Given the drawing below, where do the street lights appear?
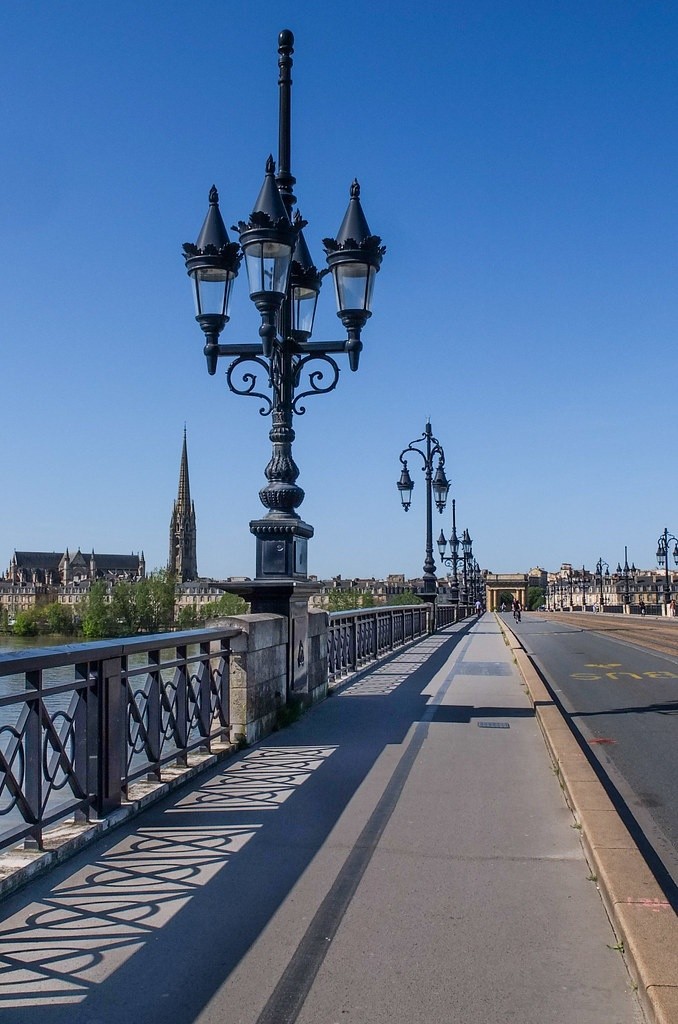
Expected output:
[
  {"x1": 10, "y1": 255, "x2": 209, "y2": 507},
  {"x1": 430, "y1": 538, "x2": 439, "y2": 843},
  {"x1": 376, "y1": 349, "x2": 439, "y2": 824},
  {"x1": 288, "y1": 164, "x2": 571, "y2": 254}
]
[
  {"x1": 656, "y1": 527, "x2": 678, "y2": 601},
  {"x1": 566, "y1": 567, "x2": 576, "y2": 605},
  {"x1": 576, "y1": 565, "x2": 592, "y2": 602},
  {"x1": 616, "y1": 546, "x2": 640, "y2": 601},
  {"x1": 595, "y1": 557, "x2": 611, "y2": 603},
  {"x1": 436, "y1": 496, "x2": 474, "y2": 599},
  {"x1": 396, "y1": 413, "x2": 453, "y2": 594},
  {"x1": 454, "y1": 528, "x2": 473, "y2": 600},
  {"x1": 180, "y1": 26, "x2": 395, "y2": 581}
]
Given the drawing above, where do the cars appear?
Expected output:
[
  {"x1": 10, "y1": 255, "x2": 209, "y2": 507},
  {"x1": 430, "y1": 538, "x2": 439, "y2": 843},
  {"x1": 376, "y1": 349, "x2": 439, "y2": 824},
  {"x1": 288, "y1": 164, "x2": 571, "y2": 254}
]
[{"x1": 537, "y1": 606, "x2": 546, "y2": 612}]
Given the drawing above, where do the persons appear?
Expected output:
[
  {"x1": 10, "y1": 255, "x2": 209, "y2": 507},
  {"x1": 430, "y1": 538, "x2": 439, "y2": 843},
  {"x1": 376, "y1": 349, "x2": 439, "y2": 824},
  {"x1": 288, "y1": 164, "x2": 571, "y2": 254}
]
[
  {"x1": 502, "y1": 603, "x2": 506, "y2": 614},
  {"x1": 474, "y1": 599, "x2": 482, "y2": 618},
  {"x1": 511, "y1": 598, "x2": 521, "y2": 622},
  {"x1": 639, "y1": 598, "x2": 646, "y2": 614},
  {"x1": 669, "y1": 600, "x2": 676, "y2": 617}
]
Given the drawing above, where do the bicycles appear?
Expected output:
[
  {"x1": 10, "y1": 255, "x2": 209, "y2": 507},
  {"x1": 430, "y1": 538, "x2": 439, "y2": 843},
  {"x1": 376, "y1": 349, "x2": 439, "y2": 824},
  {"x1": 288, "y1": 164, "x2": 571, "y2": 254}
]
[{"x1": 514, "y1": 609, "x2": 521, "y2": 624}]
